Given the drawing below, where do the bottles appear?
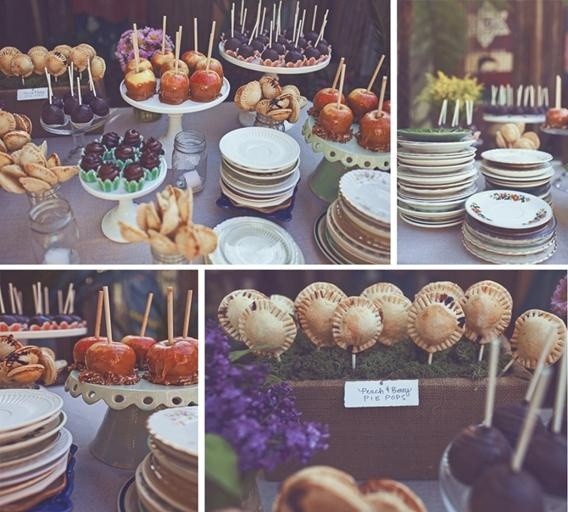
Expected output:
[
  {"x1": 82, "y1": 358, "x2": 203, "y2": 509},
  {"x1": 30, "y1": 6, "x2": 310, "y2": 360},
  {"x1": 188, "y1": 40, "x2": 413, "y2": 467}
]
[
  {"x1": 26, "y1": 196, "x2": 84, "y2": 263},
  {"x1": 171, "y1": 127, "x2": 209, "y2": 196}
]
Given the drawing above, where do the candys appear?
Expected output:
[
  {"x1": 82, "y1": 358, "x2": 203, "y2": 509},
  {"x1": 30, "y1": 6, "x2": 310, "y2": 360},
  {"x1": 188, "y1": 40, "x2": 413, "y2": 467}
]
[
  {"x1": 1, "y1": 1, "x2": 391, "y2": 263},
  {"x1": 485, "y1": 75, "x2": 568, "y2": 149},
  {"x1": 0, "y1": 282, "x2": 198, "y2": 389},
  {"x1": 215, "y1": 278, "x2": 567, "y2": 511}
]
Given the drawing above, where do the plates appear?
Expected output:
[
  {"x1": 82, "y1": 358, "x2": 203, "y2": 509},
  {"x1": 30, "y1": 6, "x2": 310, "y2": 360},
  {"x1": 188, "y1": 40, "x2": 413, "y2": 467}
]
[
  {"x1": 215, "y1": 125, "x2": 392, "y2": 264},
  {"x1": 2, "y1": 388, "x2": 202, "y2": 512},
  {"x1": 399, "y1": 126, "x2": 560, "y2": 264}
]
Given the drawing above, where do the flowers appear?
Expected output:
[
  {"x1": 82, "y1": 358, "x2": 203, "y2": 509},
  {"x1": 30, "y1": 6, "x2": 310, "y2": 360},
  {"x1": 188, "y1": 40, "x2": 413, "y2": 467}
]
[
  {"x1": 115, "y1": 27, "x2": 174, "y2": 69},
  {"x1": 205, "y1": 316, "x2": 336, "y2": 497},
  {"x1": 551, "y1": 273, "x2": 568, "y2": 316},
  {"x1": 412, "y1": 72, "x2": 485, "y2": 128}
]
